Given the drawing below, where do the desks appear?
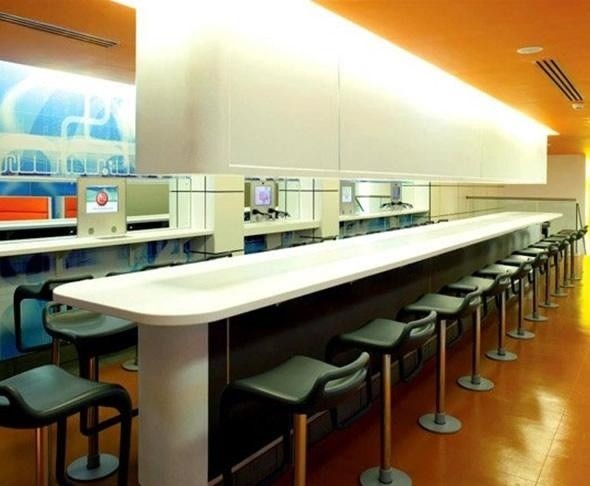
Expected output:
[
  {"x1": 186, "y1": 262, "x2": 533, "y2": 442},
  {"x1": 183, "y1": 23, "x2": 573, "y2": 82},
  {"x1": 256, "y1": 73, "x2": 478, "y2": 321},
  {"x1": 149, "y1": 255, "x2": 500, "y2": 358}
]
[{"x1": 51, "y1": 211, "x2": 566, "y2": 486}]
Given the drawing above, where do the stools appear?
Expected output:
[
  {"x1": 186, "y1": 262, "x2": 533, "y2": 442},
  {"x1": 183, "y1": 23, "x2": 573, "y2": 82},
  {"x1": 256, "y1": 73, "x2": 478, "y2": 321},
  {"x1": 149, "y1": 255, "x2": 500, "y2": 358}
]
[
  {"x1": 397, "y1": 225, "x2": 587, "y2": 434},
  {"x1": 220, "y1": 351, "x2": 371, "y2": 483},
  {"x1": 326, "y1": 311, "x2": 438, "y2": 483}
]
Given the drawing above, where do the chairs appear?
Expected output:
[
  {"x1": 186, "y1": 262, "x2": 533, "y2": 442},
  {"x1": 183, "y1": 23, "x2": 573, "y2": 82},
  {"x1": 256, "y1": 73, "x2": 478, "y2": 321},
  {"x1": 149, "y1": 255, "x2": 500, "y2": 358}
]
[{"x1": 0, "y1": 273, "x2": 140, "y2": 486}]
